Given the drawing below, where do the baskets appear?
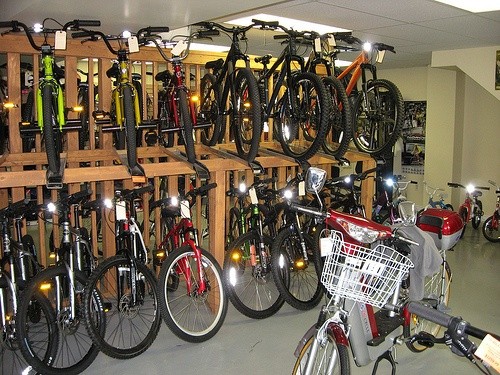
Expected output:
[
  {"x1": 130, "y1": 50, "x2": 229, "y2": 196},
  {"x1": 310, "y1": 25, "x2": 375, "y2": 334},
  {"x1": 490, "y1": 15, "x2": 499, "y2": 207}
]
[{"x1": 318, "y1": 229, "x2": 414, "y2": 309}]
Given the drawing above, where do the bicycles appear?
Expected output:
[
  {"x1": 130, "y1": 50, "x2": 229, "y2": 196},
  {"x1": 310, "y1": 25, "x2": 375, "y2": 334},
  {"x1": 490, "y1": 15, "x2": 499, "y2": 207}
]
[
  {"x1": 151, "y1": 183, "x2": 229, "y2": 343},
  {"x1": 337, "y1": 32, "x2": 405, "y2": 157},
  {"x1": 3, "y1": 165, "x2": 401, "y2": 370},
  {"x1": 222, "y1": 176, "x2": 290, "y2": 319},
  {"x1": 392, "y1": 179, "x2": 417, "y2": 201},
  {"x1": 125, "y1": 28, "x2": 221, "y2": 161},
  {"x1": 373, "y1": 175, "x2": 401, "y2": 226},
  {"x1": 483, "y1": 180, "x2": 500, "y2": 242},
  {"x1": 83, "y1": 183, "x2": 161, "y2": 360},
  {"x1": 0, "y1": 199, "x2": 59, "y2": 375},
  {"x1": 417, "y1": 181, "x2": 455, "y2": 216},
  {"x1": 255, "y1": 18, "x2": 330, "y2": 161},
  {"x1": 274, "y1": 32, "x2": 353, "y2": 157},
  {"x1": 447, "y1": 179, "x2": 490, "y2": 239},
  {"x1": 15, "y1": 188, "x2": 106, "y2": 375},
  {"x1": 195, "y1": 18, "x2": 279, "y2": 161},
  {"x1": 1, "y1": 18, "x2": 101, "y2": 174},
  {"x1": 272, "y1": 171, "x2": 326, "y2": 310},
  {"x1": 314, "y1": 167, "x2": 380, "y2": 303},
  {"x1": 70, "y1": 26, "x2": 169, "y2": 168}
]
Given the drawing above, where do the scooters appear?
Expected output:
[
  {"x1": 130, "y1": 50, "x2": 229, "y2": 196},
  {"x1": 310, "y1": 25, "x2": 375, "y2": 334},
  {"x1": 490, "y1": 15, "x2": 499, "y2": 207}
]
[
  {"x1": 290, "y1": 166, "x2": 464, "y2": 375},
  {"x1": 408, "y1": 301, "x2": 500, "y2": 375}
]
